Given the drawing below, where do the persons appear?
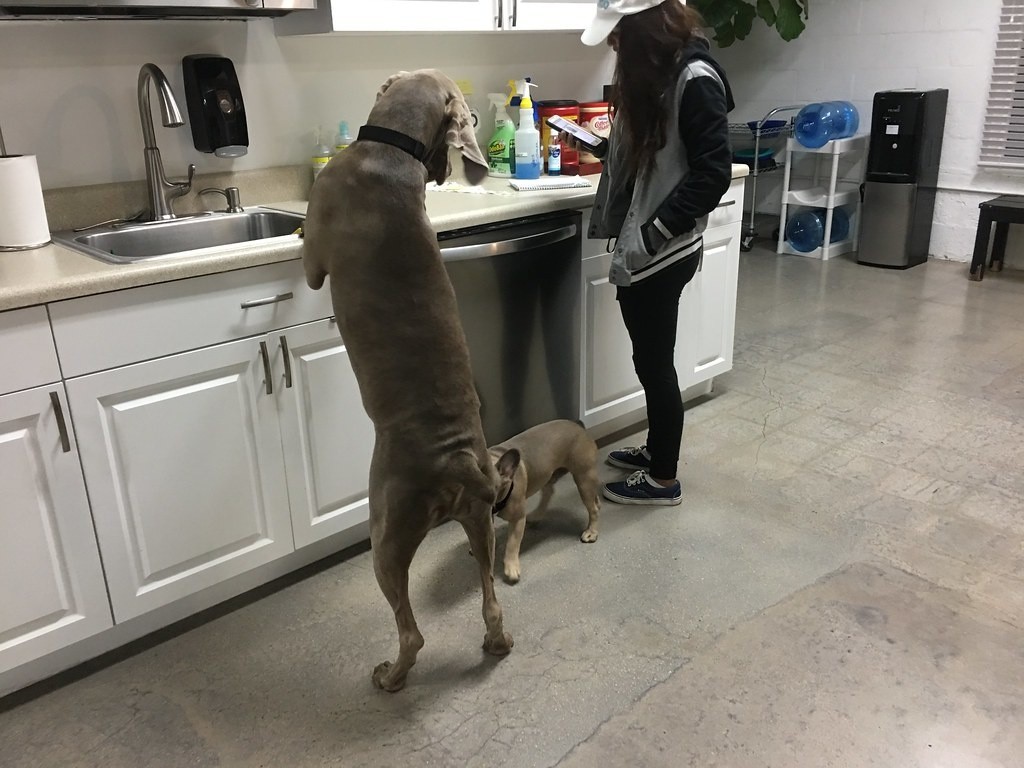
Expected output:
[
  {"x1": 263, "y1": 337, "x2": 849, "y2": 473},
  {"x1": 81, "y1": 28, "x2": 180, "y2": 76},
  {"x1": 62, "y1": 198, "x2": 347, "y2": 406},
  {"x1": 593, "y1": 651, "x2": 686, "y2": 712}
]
[{"x1": 577, "y1": 0, "x2": 734, "y2": 508}]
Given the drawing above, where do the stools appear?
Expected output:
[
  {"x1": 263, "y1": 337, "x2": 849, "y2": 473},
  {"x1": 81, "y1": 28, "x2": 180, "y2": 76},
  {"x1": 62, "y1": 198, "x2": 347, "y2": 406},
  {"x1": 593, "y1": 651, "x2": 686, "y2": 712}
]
[{"x1": 967, "y1": 192, "x2": 1024, "y2": 281}]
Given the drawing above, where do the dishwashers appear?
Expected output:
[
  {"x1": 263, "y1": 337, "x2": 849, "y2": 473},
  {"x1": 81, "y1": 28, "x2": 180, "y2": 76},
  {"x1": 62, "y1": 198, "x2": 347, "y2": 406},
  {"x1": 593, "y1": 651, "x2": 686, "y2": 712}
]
[{"x1": 428, "y1": 207, "x2": 587, "y2": 444}]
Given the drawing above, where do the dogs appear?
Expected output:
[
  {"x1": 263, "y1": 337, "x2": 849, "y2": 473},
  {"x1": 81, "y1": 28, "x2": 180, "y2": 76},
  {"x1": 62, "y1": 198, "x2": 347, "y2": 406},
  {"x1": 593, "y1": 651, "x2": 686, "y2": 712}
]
[
  {"x1": 299, "y1": 67, "x2": 519, "y2": 693},
  {"x1": 468, "y1": 418, "x2": 601, "y2": 583}
]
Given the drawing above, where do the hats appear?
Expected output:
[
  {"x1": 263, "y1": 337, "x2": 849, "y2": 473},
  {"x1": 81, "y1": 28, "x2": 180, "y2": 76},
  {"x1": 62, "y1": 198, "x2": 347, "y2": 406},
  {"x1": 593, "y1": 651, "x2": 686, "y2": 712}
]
[{"x1": 579, "y1": 1, "x2": 673, "y2": 46}]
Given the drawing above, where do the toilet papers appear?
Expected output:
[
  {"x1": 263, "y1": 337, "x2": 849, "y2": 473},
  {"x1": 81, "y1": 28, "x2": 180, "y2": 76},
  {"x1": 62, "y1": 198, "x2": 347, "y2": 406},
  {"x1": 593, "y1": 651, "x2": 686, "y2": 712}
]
[{"x1": 0, "y1": 153, "x2": 53, "y2": 253}]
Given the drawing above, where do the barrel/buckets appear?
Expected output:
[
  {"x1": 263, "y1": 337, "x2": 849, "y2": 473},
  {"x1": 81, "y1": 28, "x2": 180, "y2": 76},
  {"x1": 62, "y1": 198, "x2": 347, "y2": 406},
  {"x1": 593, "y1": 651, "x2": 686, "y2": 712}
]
[{"x1": 535, "y1": 97, "x2": 615, "y2": 170}]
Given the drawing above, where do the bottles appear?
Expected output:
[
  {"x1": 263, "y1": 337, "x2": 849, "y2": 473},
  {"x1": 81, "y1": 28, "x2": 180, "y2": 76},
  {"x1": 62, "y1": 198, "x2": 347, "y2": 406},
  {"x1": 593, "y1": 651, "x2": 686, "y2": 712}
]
[
  {"x1": 793, "y1": 100, "x2": 860, "y2": 149},
  {"x1": 787, "y1": 207, "x2": 850, "y2": 253}
]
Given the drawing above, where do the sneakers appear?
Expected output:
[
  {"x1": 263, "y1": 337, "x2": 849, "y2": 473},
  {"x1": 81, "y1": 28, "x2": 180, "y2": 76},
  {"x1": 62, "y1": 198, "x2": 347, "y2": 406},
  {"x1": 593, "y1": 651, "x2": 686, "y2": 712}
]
[
  {"x1": 599, "y1": 472, "x2": 683, "y2": 507},
  {"x1": 606, "y1": 445, "x2": 652, "y2": 471}
]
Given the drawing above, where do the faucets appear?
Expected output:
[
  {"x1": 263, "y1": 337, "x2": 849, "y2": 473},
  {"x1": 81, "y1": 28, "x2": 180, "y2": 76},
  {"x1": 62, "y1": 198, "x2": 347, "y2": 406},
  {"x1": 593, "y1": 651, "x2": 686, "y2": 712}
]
[{"x1": 136, "y1": 62, "x2": 186, "y2": 222}]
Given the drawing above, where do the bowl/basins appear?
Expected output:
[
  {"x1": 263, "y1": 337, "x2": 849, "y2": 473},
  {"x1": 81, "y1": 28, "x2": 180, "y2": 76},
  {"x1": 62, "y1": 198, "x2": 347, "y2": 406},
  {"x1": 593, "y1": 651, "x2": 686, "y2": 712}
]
[{"x1": 747, "y1": 117, "x2": 787, "y2": 138}]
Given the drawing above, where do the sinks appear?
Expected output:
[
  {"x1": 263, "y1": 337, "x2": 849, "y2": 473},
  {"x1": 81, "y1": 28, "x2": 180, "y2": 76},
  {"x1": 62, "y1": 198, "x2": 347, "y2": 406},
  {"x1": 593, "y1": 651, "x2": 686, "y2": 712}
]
[{"x1": 50, "y1": 205, "x2": 313, "y2": 265}]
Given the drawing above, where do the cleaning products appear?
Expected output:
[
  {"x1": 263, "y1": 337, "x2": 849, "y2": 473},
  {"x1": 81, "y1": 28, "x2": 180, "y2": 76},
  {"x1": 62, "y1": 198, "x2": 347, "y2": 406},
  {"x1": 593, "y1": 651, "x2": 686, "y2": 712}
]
[{"x1": 309, "y1": 77, "x2": 562, "y2": 191}]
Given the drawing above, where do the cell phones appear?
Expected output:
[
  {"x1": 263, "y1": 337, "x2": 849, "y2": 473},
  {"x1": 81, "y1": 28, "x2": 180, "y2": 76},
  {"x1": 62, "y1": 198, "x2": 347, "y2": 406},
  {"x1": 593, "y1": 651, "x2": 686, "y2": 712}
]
[{"x1": 545, "y1": 115, "x2": 602, "y2": 151}]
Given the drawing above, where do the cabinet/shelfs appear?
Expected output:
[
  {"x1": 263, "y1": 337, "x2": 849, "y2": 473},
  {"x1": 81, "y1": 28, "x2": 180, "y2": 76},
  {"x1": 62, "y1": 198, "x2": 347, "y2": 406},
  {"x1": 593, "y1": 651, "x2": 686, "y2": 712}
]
[
  {"x1": 776, "y1": 125, "x2": 870, "y2": 263},
  {"x1": 272, "y1": 2, "x2": 684, "y2": 43},
  {"x1": 725, "y1": 104, "x2": 807, "y2": 252},
  {"x1": 0, "y1": 150, "x2": 757, "y2": 707}
]
